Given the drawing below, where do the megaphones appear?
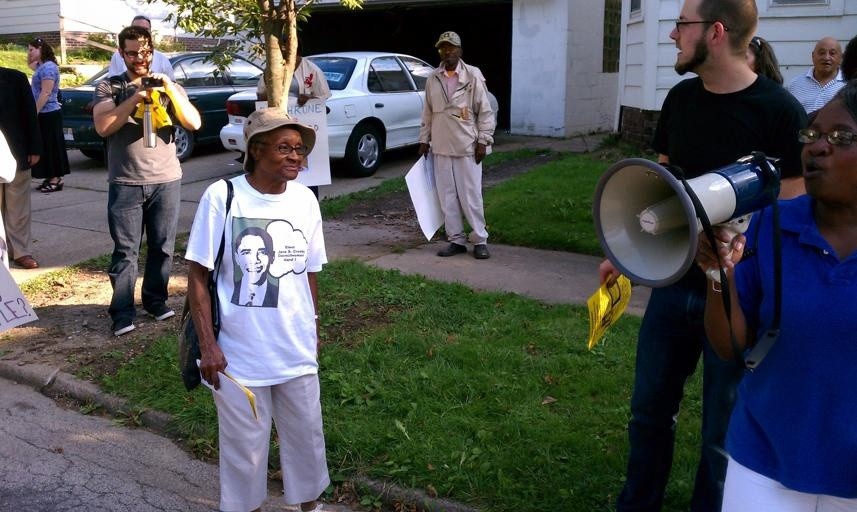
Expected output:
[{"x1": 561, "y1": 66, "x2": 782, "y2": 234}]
[{"x1": 593, "y1": 151, "x2": 781, "y2": 288}]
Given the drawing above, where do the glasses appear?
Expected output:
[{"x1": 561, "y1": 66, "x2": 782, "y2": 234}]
[
  {"x1": 255, "y1": 139, "x2": 308, "y2": 156},
  {"x1": 797, "y1": 127, "x2": 857, "y2": 149},
  {"x1": 121, "y1": 48, "x2": 154, "y2": 58},
  {"x1": 438, "y1": 47, "x2": 460, "y2": 54},
  {"x1": 674, "y1": 17, "x2": 729, "y2": 34}
]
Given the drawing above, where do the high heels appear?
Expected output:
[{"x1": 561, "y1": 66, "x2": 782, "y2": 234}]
[
  {"x1": 41, "y1": 178, "x2": 64, "y2": 193},
  {"x1": 35, "y1": 179, "x2": 51, "y2": 191}
]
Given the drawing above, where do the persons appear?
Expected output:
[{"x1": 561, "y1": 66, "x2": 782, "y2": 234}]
[
  {"x1": 27, "y1": 37, "x2": 71, "y2": 193},
  {"x1": 418, "y1": 30, "x2": 497, "y2": 258},
  {"x1": 600, "y1": 2, "x2": 808, "y2": 512},
  {"x1": 182, "y1": 106, "x2": 332, "y2": 512},
  {"x1": 0, "y1": 66, "x2": 43, "y2": 269},
  {"x1": 91, "y1": 26, "x2": 203, "y2": 336},
  {"x1": 698, "y1": 87, "x2": 856, "y2": 511},
  {"x1": 746, "y1": 36, "x2": 856, "y2": 119},
  {"x1": 231, "y1": 226, "x2": 279, "y2": 307},
  {"x1": 256, "y1": 31, "x2": 332, "y2": 202},
  {"x1": 108, "y1": 16, "x2": 176, "y2": 82},
  {"x1": 0, "y1": 129, "x2": 17, "y2": 269}
]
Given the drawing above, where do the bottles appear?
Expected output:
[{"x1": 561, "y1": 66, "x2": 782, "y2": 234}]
[{"x1": 142, "y1": 96, "x2": 157, "y2": 148}]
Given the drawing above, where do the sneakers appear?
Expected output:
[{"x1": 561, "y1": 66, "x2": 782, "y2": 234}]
[
  {"x1": 472, "y1": 243, "x2": 491, "y2": 259},
  {"x1": 144, "y1": 302, "x2": 176, "y2": 321},
  {"x1": 110, "y1": 318, "x2": 137, "y2": 337},
  {"x1": 436, "y1": 242, "x2": 468, "y2": 257}
]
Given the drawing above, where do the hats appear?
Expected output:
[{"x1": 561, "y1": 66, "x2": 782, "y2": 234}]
[
  {"x1": 241, "y1": 106, "x2": 316, "y2": 174},
  {"x1": 435, "y1": 31, "x2": 462, "y2": 48}
]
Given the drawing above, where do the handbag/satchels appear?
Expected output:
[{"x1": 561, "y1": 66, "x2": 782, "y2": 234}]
[{"x1": 177, "y1": 276, "x2": 223, "y2": 393}]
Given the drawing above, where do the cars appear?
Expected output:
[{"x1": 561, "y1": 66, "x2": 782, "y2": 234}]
[
  {"x1": 54, "y1": 47, "x2": 264, "y2": 164},
  {"x1": 218, "y1": 50, "x2": 502, "y2": 180}
]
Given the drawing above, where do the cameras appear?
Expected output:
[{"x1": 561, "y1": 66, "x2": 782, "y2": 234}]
[{"x1": 142, "y1": 77, "x2": 163, "y2": 87}]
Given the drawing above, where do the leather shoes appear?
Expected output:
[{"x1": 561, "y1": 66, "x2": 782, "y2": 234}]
[{"x1": 14, "y1": 255, "x2": 39, "y2": 270}]
